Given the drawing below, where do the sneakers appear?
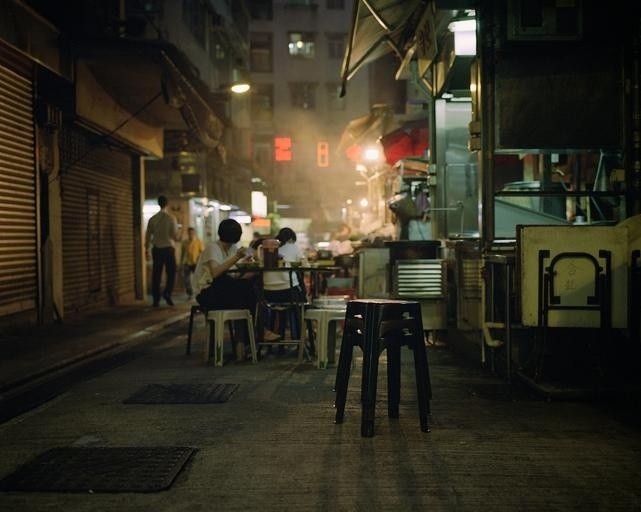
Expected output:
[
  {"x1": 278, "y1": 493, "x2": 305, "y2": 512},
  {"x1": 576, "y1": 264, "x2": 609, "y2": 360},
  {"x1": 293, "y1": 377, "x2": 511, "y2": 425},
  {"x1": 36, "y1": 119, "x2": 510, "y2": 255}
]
[
  {"x1": 257, "y1": 326, "x2": 281, "y2": 340},
  {"x1": 153, "y1": 291, "x2": 174, "y2": 306}
]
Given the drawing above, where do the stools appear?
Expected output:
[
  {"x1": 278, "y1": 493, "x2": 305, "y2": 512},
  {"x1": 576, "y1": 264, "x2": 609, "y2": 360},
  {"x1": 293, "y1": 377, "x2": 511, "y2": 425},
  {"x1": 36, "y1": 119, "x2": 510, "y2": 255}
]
[
  {"x1": 188, "y1": 294, "x2": 348, "y2": 371},
  {"x1": 332, "y1": 300, "x2": 434, "y2": 439}
]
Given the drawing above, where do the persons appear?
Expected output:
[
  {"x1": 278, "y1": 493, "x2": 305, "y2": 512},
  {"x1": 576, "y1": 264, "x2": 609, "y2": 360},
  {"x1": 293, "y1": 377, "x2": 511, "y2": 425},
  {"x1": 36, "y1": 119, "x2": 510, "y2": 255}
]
[
  {"x1": 179, "y1": 226, "x2": 202, "y2": 302},
  {"x1": 145, "y1": 195, "x2": 183, "y2": 308},
  {"x1": 191, "y1": 219, "x2": 304, "y2": 343},
  {"x1": 329, "y1": 224, "x2": 354, "y2": 260}
]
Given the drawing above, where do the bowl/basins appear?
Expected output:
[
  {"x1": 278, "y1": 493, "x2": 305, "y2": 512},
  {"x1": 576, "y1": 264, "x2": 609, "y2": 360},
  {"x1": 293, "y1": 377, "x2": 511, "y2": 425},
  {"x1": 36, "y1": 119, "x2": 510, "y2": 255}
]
[
  {"x1": 234, "y1": 262, "x2": 259, "y2": 270},
  {"x1": 289, "y1": 261, "x2": 301, "y2": 268}
]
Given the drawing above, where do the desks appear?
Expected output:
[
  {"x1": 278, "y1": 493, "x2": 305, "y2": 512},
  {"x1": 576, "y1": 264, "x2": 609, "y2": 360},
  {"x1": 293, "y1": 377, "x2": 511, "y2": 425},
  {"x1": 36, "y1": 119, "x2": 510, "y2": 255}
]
[{"x1": 228, "y1": 266, "x2": 336, "y2": 362}]
